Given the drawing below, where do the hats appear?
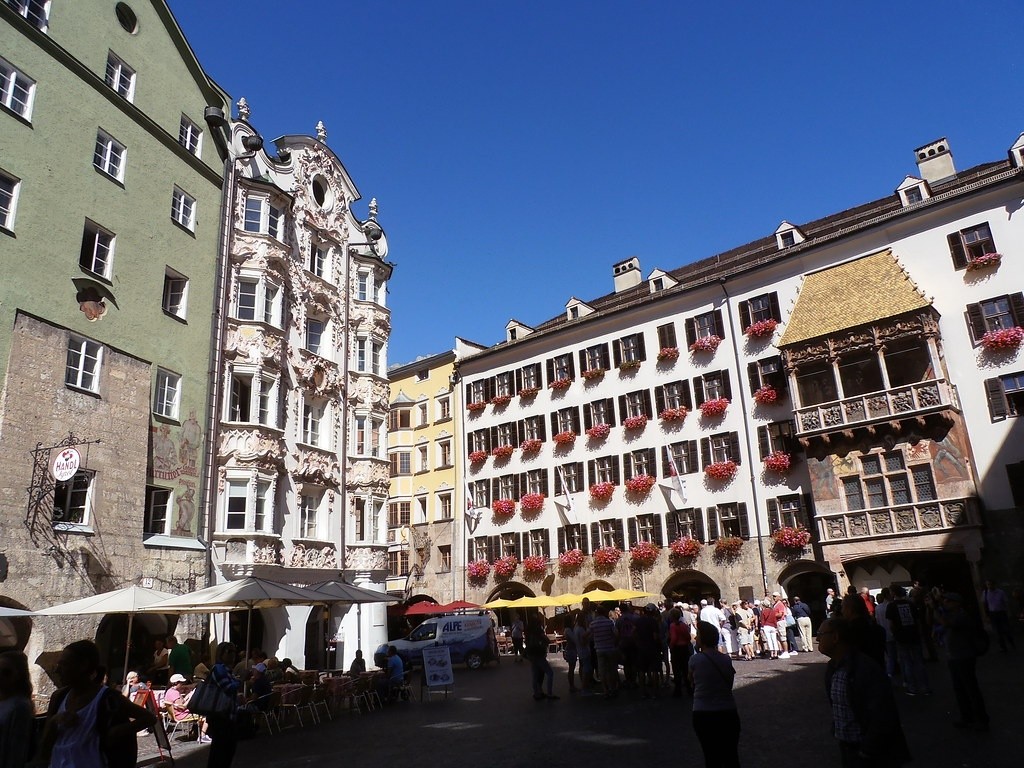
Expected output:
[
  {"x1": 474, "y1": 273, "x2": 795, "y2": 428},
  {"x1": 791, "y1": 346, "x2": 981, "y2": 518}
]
[
  {"x1": 761, "y1": 600, "x2": 771, "y2": 607},
  {"x1": 252, "y1": 662, "x2": 267, "y2": 672},
  {"x1": 772, "y1": 592, "x2": 780, "y2": 596},
  {"x1": 170, "y1": 674, "x2": 187, "y2": 684},
  {"x1": 677, "y1": 601, "x2": 682, "y2": 606},
  {"x1": 701, "y1": 599, "x2": 707, "y2": 605}
]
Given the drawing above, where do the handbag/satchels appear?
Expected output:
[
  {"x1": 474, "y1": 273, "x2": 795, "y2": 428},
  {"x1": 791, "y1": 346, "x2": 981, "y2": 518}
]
[
  {"x1": 786, "y1": 616, "x2": 796, "y2": 626},
  {"x1": 983, "y1": 590, "x2": 990, "y2": 617},
  {"x1": 792, "y1": 627, "x2": 800, "y2": 637},
  {"x1": 523, "y1": 646, "x2": 543, "y2": 663},
  {"x1": 722, "y1": 635, "x2": 726, "y2": 642},
  {"x1": 688, "y1": 640, "x2": 694, "y2": 655},
  {"x1": 47, "y1": 685, "x2": 109, "y2": 768},
  {"x1": 188, "y1": 666, "x2": 232, "y2": 717}
]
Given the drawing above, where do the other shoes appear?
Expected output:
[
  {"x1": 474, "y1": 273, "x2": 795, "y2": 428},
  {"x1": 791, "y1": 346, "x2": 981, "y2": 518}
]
[
  {"x1": 136, "y1": 728, "x2": 149, "y2": 736},
  {"x1": 769, "y1": 657, "x2": 775, "y2": 660},
  {"x1": 903, "y1": 680, "x2": 908, "y2": 687},
  {"x1": 529, "y1": 676, "x2": 693, "y2": 700},
  {"x1": 904, "y1": 690, "x2": 916, "y2": 695},
  {"x1": 743, "y1": 656, "x2": 755, "y2": 661},
  {"x1": 774, "y1": 656, "x2": 778, "y2": 658}
]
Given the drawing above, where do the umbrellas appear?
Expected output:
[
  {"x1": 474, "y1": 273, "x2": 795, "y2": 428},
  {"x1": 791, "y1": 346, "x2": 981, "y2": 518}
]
[
  {"x1": 0, "y1": 603, "x2": 46, "y2": 622},
  {"x1": 297, "y1": 580, "x2": 405, "y2": 668},
  {"x1": 389, "y1": 587, "x2": 658, "y2": 624},
  {"x1": 35, "y1": 584, "x2": 243, "y2": 686},
  {"x1": 139, "y1": 575, "x2": 349, "y2": 669}
]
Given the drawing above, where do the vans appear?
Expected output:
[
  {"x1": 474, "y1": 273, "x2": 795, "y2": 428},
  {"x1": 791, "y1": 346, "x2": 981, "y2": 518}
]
[{"x1": 374, "y1": 614, "x2": 501, "y2": 672}]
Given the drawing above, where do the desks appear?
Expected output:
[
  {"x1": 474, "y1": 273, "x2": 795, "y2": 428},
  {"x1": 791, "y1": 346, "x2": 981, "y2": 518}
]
[
  {"x1": 152, "y1": 690, "x2": 166, "y2": 707},
  {"x1": 358, "y1": 670, "x2": 385, "y2": 689},
  {"x1": 297, "y1": 670, "x2": 319, "y2": 682},
  {"x1": 272, "y1": 683, "x2": 302, "y2": 729},
  {"x1": 326, "y1": 675, "x2": 351, "y2": 686}
]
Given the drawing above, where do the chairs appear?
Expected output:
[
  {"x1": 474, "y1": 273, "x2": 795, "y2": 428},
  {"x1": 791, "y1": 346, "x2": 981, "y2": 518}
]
[
  {"x1": 495, "y1": 635, "x2": 514, "y2": 655},
  {"x1": 156, "y1": 670, "x2": 418, "y2": 744},
  {"x1": 547, "y1": 634, "x2": 560, "y2": 654}
]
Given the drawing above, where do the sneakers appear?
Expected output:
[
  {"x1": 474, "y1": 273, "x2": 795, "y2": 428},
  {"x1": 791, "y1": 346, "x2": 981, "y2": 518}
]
[
  {"x1": 197, "y1": 735, "x2": 212, "y2": 742},
  {"x1": 778, "y1": 653, "x2": 790, "y2": 658},
  {"x1": 789, "y1": 651, "x2": 798, "y2": 656}
]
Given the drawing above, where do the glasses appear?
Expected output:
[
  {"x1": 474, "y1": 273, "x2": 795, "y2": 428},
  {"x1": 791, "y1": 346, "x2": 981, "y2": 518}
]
[{"x1": 816, "y1": 630, "x2": 835, "y2": 638}]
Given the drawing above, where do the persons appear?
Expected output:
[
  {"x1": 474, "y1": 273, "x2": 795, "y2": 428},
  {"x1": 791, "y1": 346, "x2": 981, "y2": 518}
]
[
  {"x1": 511, "y1": 614, "x2": 525, "y2": 661},
  {"x1": 526, "y1": 613, "x2": 562, "y2": 701},
  {"x1": 381, "y1": 646, "x2": 413, "y2": 705},
  {"x1": 234, "y1": 652, "x2": 298, "y2": 738},
  {"x1": 349, "y1": 649, "x2": 365, "y2": 672},
  {"x1": 676, "y1": 592, "x2": 814, "y2": 659},
  {"x1": 687, "y1": 621, "x2": 740, "y2": 768},
  {"x1": 0, "y1": 647, "x2": 139, "y2": 768},
  {"x1": 816, "y1": 620, "x2": 913, "y2": 768},
  {"x1": 826, "y1": 586, "x2": 900, "y2": 668},
  {"x1": 564, "y1": 597, "x2": 694, "y2": 701},
  {"x1": 910, "y1": 579, "x2": 1024, "y2": 730},
  {"x1": 114, "y1": 635, "x2": 212, "y2": 743},
  {"x1": 885, "y1": 588, "x2": 930, "y2": 695},
  {"x1": 203, "y1": 643, "x2": 239, "y2": 768}
]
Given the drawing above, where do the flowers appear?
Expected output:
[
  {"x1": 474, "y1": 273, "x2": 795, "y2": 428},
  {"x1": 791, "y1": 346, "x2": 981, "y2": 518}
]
[
  {"x1": 981, "y1": 326, "x2": 1024, "y2": 352},
  {"x1": 966, "y1": 252, "x2": 1005, "y2": 272},
  {"x1": 466, "y1": 317, "x2": 812, "y2": 583}
]
